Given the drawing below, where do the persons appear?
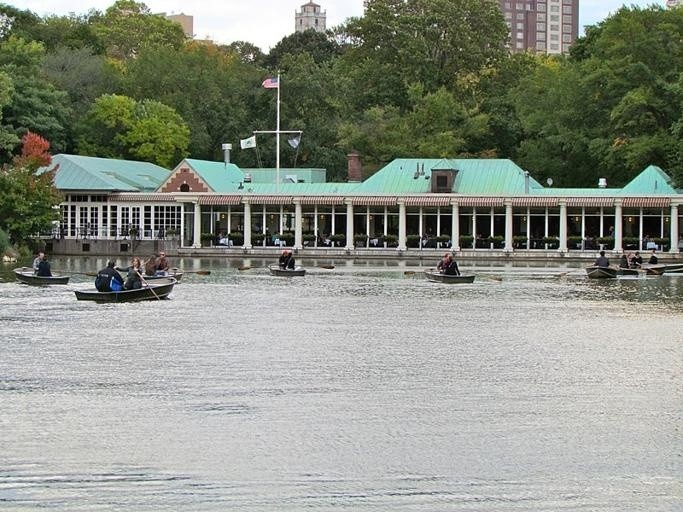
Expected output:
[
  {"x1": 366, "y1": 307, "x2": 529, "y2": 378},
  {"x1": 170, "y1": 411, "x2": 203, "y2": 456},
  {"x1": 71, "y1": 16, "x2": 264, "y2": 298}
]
[
  {"x1": 113, "y1": 256, "x2": 143, "y2": 289},
  {"x1": 617, "y1": 250, "x2": 642, "y2": 269},
  {"x1": 144, "y1": 255, "x2": 156, "y2": 276},
  {"x1": 283, "y1": 252, "x2": 294, "y2": 270},
  {"x1": 592, "y1": 250, "x2": 610, "y2": 268},
  {"x1": 436, "y1": 253, "x2": 460, "y2": 276},
  {"x1": 277, "y1": 250, "x2": 287, "y2": 267},
  {"x1": 95, "y1": 258, "x2": 124, "y2": 292},
  {"x1": 154, "y1": 251, "x2": 169, "y2": 271},
  {"x1": 36, "y1": 256, "x2": 52, "y2": 276},
  {"x1": 646, "y1": 249, "x2": 657, "y2": 264},
  {"x1": 31, "y1": 251, "x2": 44, "y2": 273}
]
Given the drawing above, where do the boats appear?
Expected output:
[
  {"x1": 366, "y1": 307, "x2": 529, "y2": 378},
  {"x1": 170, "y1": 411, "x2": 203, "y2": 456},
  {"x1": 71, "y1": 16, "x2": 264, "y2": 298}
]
[
  {"x1": 266, "y1": 266, "x2": 306, "y2": 276},
  {"x1": 141, "y1": 273, "x2": 183, "y2": 282},
  {"x1": 586, "y1": 264, "x2": 683, "y2": 278},
  {"x1": 425, "y1": 271, "x2": 475, "y2": 283},
  {"x1": 12, "y1": 266, "x2": 69, "y2": 285},
  {"x1": 73, "y1": 280, "x2": 178, "y2": 303}
]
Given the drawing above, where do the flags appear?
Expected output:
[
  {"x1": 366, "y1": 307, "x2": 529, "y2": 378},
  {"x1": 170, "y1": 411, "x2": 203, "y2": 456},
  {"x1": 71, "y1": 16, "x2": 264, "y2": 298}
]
[
  {"x1": 287, "y1": 134, "x2": 303, "y2": 149},
  {"x1": 260, "y1": 76, "x2": 278, "y2": 89},
  {"x1": 239, "y1": 135, "x2": 256, "y2": 150}
]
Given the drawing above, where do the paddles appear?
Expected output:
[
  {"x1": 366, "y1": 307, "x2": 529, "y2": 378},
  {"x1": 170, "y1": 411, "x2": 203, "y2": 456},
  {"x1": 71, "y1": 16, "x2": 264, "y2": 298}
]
[
  {"x1": 137, "y1": 271, "x2": 162, "y2": 300},
  {"x1": 51, "y1": 270, "x2": 98, "y2": 277},
  {"x1": 293, "y1": 265, "x2": 334, "y2": 269},
  {"x1": 639, "y1": 264, "x2": 661, "y2": 275},
  {"x1": 238, "y1": 265, "x2": 278, "y2": 270},
  {"x1": 167, "y1": 271, "x2": 211, "y2": 275},
  {"x1": 404, "y1": 271, "x2": 441, "y2": 275},
  {"x1": 555, "y1": 264, "x2": 594, "y2": 276},
  {"x1": 460, "y1": 271, "x2": 503, "y2": 281}
]
